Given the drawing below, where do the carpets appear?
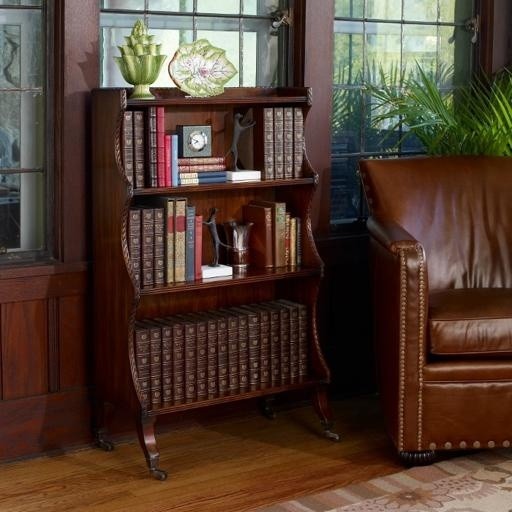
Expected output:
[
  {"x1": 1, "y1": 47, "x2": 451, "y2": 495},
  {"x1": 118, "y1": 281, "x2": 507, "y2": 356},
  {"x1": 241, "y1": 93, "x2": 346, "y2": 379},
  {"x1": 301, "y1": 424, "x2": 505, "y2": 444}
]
[{"x1": 256, "y1": 451, "x2": 512, "y2": 512}]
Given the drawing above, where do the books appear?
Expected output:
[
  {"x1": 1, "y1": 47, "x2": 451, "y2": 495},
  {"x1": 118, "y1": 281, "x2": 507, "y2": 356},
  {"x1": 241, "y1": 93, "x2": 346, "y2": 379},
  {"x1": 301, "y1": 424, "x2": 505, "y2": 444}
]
[
  {"x1": 134, "y1": 299, "x2": 307, "y2": 410},
  {"x1": 128, "y1": 196, "x2": 302, "y2": 288},
  {"x1": 122, "y1": 107, "x2": 303, "y2": 188}
]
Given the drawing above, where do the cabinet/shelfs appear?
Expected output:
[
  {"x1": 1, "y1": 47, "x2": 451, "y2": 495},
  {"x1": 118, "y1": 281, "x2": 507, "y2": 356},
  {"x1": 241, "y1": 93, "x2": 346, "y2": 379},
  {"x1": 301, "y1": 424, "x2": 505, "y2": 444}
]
[{"x1": 90, "y1": 87, "x2": 342, "y2": 481}]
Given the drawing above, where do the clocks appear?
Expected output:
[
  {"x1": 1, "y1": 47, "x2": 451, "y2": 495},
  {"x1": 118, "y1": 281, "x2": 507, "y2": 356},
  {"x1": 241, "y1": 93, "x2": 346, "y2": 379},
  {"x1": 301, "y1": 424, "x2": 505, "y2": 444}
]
[{"x1": 176, "y1": 125, "x2": 212, "y2": 158}]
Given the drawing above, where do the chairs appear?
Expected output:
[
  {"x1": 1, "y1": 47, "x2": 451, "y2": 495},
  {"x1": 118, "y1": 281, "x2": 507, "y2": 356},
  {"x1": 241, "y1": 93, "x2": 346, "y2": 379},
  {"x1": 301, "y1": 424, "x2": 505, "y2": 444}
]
[{"x1": 355, "y1": 155, "x2": 512, "y2": 466}]
